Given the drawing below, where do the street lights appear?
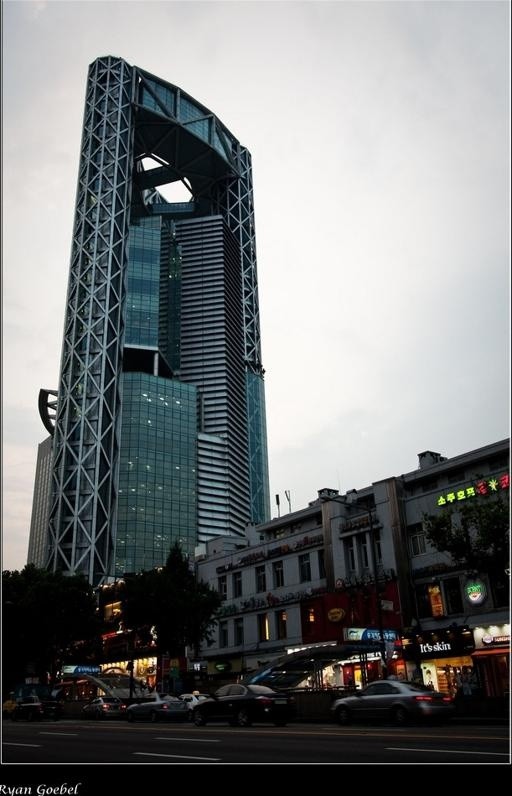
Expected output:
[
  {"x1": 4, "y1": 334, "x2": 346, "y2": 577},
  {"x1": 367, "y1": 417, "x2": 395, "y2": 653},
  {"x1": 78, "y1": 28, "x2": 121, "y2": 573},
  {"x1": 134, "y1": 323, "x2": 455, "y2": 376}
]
[
  {"x1": 95, "y1": 572, "x2": 136, "y2": 698},
  {"x1": 320, "y1": 495, "x2": 388, "y2": 679}
]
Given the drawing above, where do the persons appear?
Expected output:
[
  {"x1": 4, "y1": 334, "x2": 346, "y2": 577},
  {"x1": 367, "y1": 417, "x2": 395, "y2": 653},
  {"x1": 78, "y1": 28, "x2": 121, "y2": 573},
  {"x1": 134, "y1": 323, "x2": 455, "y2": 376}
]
[{"x1": 178, "y1": 691, "x2": 201, "y2": 724}]
